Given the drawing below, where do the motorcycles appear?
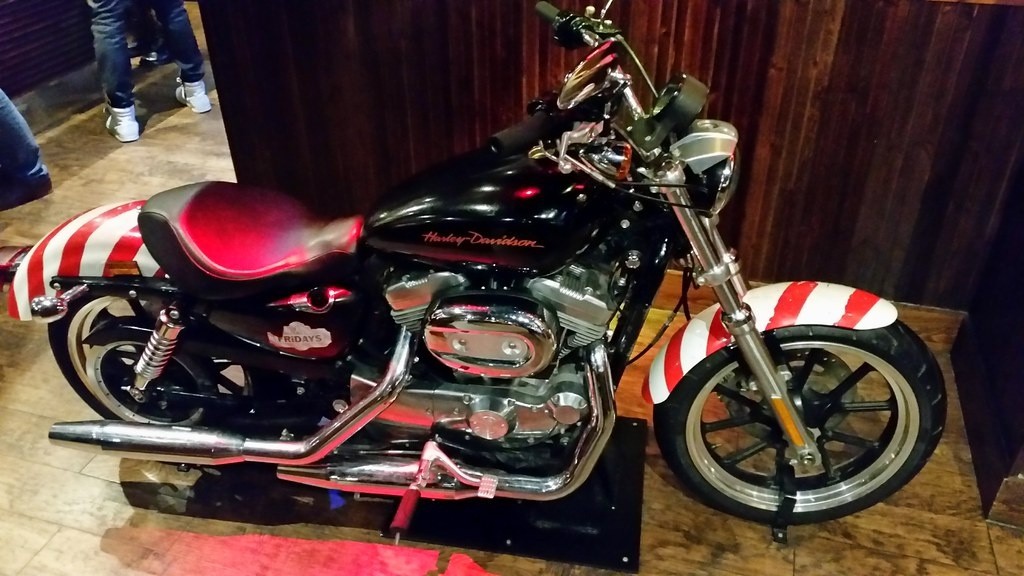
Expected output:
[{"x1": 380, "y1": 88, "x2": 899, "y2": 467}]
[{"x1": 1, "y1": 0, "x2": 947, "y2": 545}]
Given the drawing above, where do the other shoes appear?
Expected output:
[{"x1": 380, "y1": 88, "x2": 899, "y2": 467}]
[{"x1": 0, "y1": 174, "x2": 52, "y2": 211}]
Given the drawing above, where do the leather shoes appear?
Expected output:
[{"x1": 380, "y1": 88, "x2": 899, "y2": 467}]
[
  {"x1": 127, "y1": 38, "x2": 149, "y2": 58},
  {"x1": 138, "y1": 45, "x2": 172, "y2": 66}
]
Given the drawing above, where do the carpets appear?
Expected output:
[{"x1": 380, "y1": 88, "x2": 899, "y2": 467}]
[{"x1": 381, "y1": 415, "x2": 647, "y2": 575}]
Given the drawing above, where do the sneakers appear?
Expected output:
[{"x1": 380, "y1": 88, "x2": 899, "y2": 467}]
[
  {"x1": 174, "y1": 75, "x2": 213, "y2": 113},
  {"x1": 103, "y1": 100, "x2": 140, "y2": 142}
]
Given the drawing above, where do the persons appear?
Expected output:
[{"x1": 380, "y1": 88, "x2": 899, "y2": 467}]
[
  {"x1": 85, "y1": 0, "x2": 213, "y2": 143},
  {"x1": 0, "y1": 89, "x2": 53, "y2": 211}
]
